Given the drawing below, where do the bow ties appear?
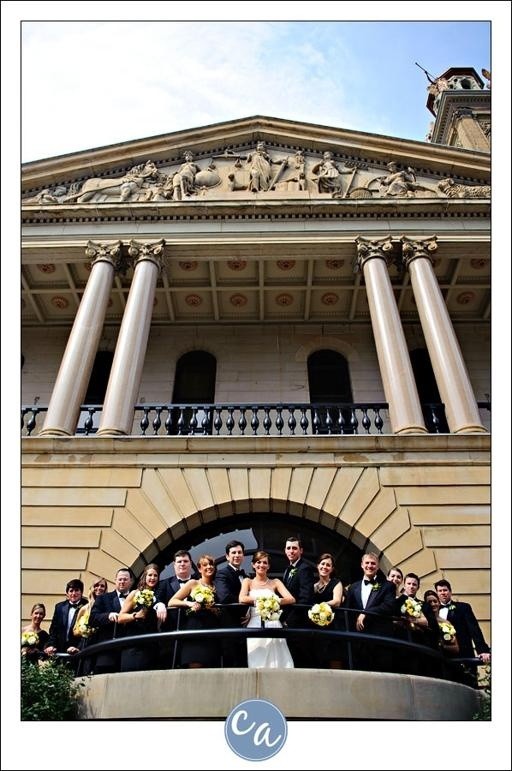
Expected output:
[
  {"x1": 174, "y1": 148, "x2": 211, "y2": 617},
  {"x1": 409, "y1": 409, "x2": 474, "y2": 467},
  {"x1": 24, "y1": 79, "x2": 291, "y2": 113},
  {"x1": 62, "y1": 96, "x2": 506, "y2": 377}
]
[
  {"x1": 119, "y1": 593, "x2": 128, "y2": 599},
  {"x1": 69, "y1": 601, "x2": 81, "y2": 608},
  {"x1": 235, "y1": 569, "x2": 245, "y2": 576},
  {"x1": 178, "y1": 579, "x2": 188, "y2": 583},
  {"x1": 440, "y1": 604, "x2": 450, "y2": 608},
  {"x1": 364, "y1": 578, "x2": 375, "y2": 585}
]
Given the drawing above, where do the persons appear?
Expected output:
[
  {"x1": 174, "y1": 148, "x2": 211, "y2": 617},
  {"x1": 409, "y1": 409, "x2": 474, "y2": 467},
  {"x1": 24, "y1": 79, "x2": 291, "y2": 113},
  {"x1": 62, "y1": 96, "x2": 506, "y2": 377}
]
[
  {"x1": 33, "y1": 139, "x2": 416, "y2": 205},
  {"x1": 20, "y1": 537, "x2": 490, "y2": 689}
]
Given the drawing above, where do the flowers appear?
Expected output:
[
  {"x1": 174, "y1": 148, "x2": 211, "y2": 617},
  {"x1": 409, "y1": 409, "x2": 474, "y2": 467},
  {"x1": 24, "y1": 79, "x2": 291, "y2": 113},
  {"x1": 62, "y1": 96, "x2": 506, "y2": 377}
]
[
  {"x1": 192, "y1": 584, "x2": 216, "y2": 606},
  {"x1": 254, "y1": 594, "x2": 282, "y2": 621},
  {"x1": 22, "y1": 631, "x2": 38, "y2": 645},
  {"x1": 286, "y1": 567, "x2": 298, "y2": 576},
  {"x1": 372, "y1": 580, "x2": 381, "y2": 591},
  {"x1": 307, "y1": 601, "x2": 334, "y2": 627},
  {"x1": 73, "y1": 614, "x2": 94, "y2": 637},
  {"x1": 401, "y1": 596, "x2": 458, "y2": 645},
  {"x1": 133, "y1": 588, "x2": 155, "y2": 606}
]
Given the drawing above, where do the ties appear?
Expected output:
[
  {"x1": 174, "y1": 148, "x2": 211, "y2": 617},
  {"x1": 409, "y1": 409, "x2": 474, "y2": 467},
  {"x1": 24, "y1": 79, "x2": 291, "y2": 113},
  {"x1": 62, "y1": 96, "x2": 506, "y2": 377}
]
[{"x1": 283, "y1": 565, "x2": 295, "y2": 586}]
